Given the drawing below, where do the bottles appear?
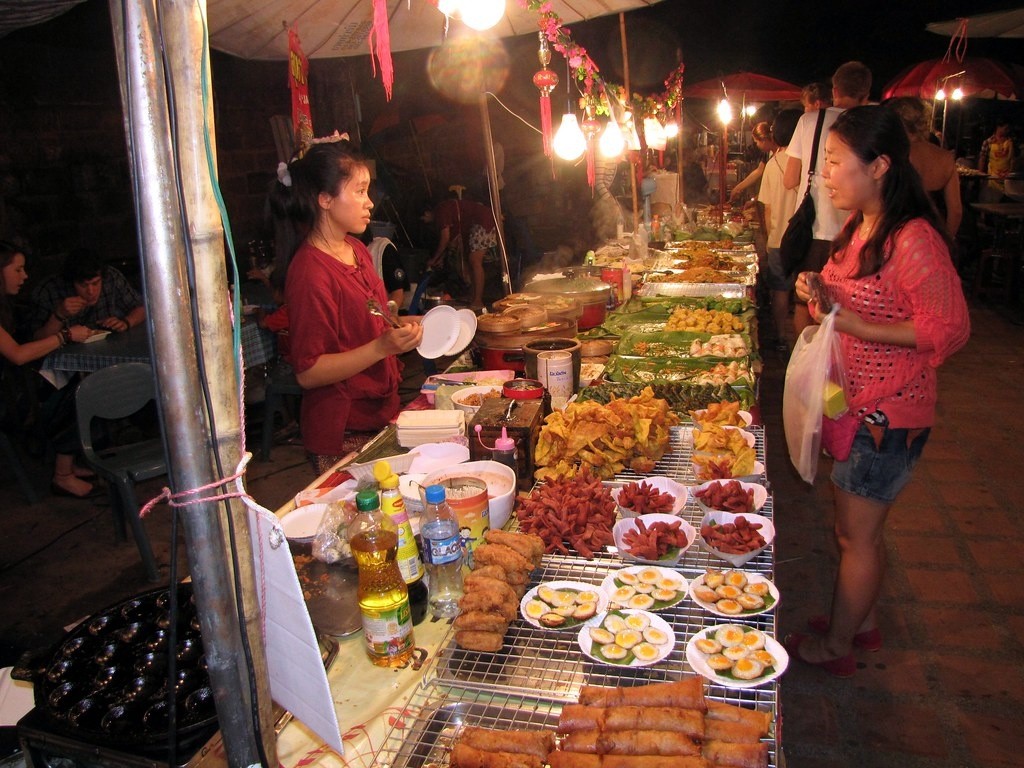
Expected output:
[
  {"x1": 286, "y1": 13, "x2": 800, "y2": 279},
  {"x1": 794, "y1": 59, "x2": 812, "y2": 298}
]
[
  {"x1": 536, "y1": 351, "x2": 573, "y2": 398},
  {"x1": 521, "y1": 338, "x2": 580, "y2": 424},
  {"x1": 374, "y1": 460, "x2": 424, "y2": 586},
  {"x1": 419, "y1": 477, "x2": 490, "y2": 577},
  {"x1": 420, "y1": 484, "x2": 464, "y2": 617},
  {"x1": 636, "y1": 223, "x2": 648, "y2": 246},
  {"x1": 623, "y1": 263, "x2": 631, "y2": 299},
  {"x1": 475, "y1": 424, "x2": 519, "y2": 497},
  {"x1": 650, "y1": 214, "x2": 660, "y2": 242},
  {"x1": 349, "y1": 490, "x2": 414, "y2": 667},
  {"x1": 600, "y1": 268, "x2": 622, "y2": 311}
]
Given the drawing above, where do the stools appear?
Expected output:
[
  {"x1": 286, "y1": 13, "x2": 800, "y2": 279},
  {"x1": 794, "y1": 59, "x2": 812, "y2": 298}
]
[{"x1": 396, "y1": 246, "x2": 438, "y2": 288}]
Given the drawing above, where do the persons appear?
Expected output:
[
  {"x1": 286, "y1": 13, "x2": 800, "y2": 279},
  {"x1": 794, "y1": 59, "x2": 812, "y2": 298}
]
[
  {"x1": 729, "y1": 104, "x2": 799, "y2": 353},
  {"x1": 247, "y1": 212, "x2": 312, "y2": 443},
  {"x1": 591, "y1": 160, "x2": 628, "y2": 247},
  {"x1": 978, "y1": 118, "x2": 1015, "y2": 200},
  {"x1": 32, "y1": 247, "x2": 147, "y2": 342},
  {"x1": 783, "y1": 103, "x2": 968, "y2": 678},
  {"x1": 784, "y1": 61, "x2": 873, "y2": 336},
  {"x1": 347, "y1": 225, "x2": 411, "y2": 322},
  {"x1": 0, "y1": 242, "x2": 110, "y2": 500},
  {"x1": 415, "y1": 197, "x2": 503, "y2": 310},
  {"x1": 282, "y1": 139, "x2": 429, "y2": 476},
  {"x1": 879, "y1": 97, "x2": 962, "y2": 248}
]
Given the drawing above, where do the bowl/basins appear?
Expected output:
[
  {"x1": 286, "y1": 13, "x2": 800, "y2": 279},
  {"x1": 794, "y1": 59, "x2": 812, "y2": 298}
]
[
  {"x1": 689, "y1": 407, "x2": 765, "y2": 485},
  {"x1": 610, "y1": 475, "x2": 776, "y2": 567},
  {"x1": 418, "y1": 460, "x2": 516, "y2": 529},
  {"x1": 339, "y1": 452, "x2": 420, "y2": 482},
  {"x1": 280, "y1": 503, "x2": 328, "y2": 543},
  {"x1": 451, "y1": 385, "x2": 504, "y2": 415},
  {"x1": 555, "y1": 257, "x2": 622, "y2": 310}
]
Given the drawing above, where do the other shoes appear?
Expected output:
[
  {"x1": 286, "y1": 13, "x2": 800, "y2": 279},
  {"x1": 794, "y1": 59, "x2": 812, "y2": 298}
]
[
  {"x1": 470, "y1": 303, "x2": 485, "y2": 311},
  {"x1": 783, "y1": 633, "x2": 857, "y2": 679},
  {"x1": 458, "y1": 297, "x2": 471, "y2": 304},
  {"x1": 273, "y1": 420, "x2": 300, "y2": 442},
  {"x1": 811, "y1": 613, "x2": 882, "y2": 652},
  {"x1": 48, "y1": 464, "x2": 101, "y2": 499}
]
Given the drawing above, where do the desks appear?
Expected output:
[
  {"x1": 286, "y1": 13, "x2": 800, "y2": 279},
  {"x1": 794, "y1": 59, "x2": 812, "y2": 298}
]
[{"x1": 31, "y1": 314, "x2": 277, "y2": 473}]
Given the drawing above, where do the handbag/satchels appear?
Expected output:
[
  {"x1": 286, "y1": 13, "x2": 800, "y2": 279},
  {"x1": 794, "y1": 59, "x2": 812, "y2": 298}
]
[
  {"x1": 450, "y1": 233, "x2": 471, "y2": 288},
  {"x1": 779, "y1": 195, "x2": 815, "y2": 279},
  {"x1": 822, "y1": 408, "x2": 868, "y2": 462},
  {"x1": 782, "y1": 305, "x2": 840, "y2": 483}
]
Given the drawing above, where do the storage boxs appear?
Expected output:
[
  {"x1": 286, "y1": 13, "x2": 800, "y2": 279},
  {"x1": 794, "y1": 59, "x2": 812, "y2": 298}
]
[{"x1": 469, "y1": 399, "x2": 545, "y2": 492}]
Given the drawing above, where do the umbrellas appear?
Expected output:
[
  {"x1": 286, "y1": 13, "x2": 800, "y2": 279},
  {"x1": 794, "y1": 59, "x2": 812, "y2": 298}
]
[
  {"x1": 680, "y1": 70, "x2": 803, "y2": 151},
  {"x1": 927, "y1": 8, "x2": 1024, "y2": 38},
  {"x1": 207, "y1": 0, "x2": 664, "y2": 64},
  {"x1": 887, "y1": 52, "x2": 1017, "y2": 143}
]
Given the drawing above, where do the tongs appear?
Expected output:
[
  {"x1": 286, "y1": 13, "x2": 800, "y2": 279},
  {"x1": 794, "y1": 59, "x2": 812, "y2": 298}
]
[{"x1": 366, "y1": 297, "x2": 404, "y2": 329}]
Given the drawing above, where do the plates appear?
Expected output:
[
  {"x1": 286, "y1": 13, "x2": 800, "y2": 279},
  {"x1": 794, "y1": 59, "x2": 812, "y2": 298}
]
[
  {"x1": 443, "y1": 309, "x2": 477, "y2": 355},
  {"x1": 417, "y1": 305, "x2": 460, "y2": 359},
  {"x1": 398, "y1": 474, "x2": 424, "y2": 512},
  {"x1": 578, "y1": 565, "x2": 789, "y2": 688},
  {"x1": 403, "y1": 442, "x2": 470, "y2": 473},
  {"x1": 397, "y1": 410, "x2": 465, "y2": 450},
  {"x1": 519, "y1": 580, "x2": 609, "y2": 629}
]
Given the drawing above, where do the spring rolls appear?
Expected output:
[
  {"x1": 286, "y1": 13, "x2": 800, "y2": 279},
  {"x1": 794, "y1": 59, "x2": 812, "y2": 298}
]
[{"x1": 445, "y1": 673, "x2": 772, "y2": 768}]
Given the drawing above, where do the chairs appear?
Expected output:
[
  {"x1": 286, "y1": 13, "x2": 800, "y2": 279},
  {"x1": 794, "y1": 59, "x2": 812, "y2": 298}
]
[
  {"x1": 75, "y1": 362, "x2": 167, "y2": 582},
  {"x1": 259, "y1": 372, "x2": 304, "y2": 462},
  {"x1": 0, "y1": 397, "x2": 39, "y2": 506}
]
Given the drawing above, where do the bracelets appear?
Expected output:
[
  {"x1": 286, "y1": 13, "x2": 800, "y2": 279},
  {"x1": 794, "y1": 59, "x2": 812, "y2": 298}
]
[
  {"x1": 121, "y1": 317, "x2": 130, "y2": 332},
  {"x1": 54, "y1": 327, "x2": 72, "y2": 347},
  {"x1": 54, "y1": 310, "x2": 66, "y2": 322}
]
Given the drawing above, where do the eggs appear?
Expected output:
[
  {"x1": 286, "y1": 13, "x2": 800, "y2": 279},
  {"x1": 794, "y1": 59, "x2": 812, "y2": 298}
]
[{"x1": 526, "y1": 567, "x2": 777, "y2": 679}]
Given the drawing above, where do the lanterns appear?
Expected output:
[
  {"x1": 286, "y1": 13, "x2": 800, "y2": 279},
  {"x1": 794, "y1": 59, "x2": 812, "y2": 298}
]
[{"x1": 532, "y1": 66, "x2": 682, "y2": 193}]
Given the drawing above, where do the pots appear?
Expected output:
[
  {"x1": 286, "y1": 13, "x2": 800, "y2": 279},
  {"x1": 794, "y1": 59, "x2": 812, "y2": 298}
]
[
  {"x1": 475, "y1": 314, "x2": 578, "y2": 379},
  {"x1": 526, "y1": 269, "x2": 615, "y2": 330}
]
